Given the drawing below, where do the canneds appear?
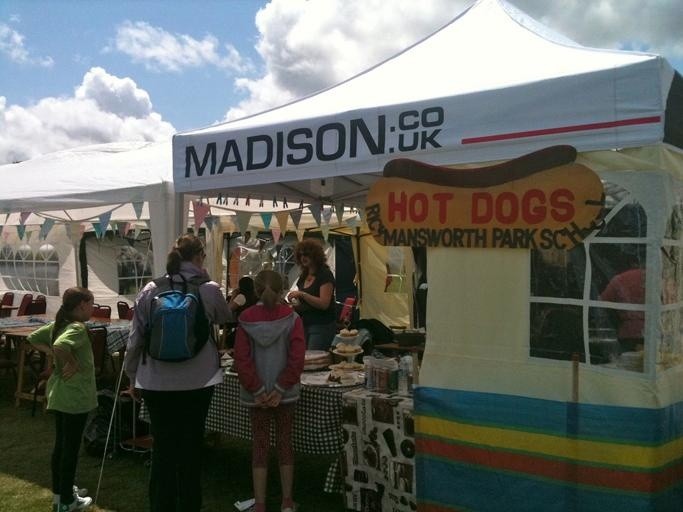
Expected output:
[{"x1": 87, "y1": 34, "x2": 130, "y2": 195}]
[
  {"x1": 387, "y1": 368, "x2": 398, "y2": 394},
  {"x1": 378, "y1": 367, "x2": 389, "y2": 393}
]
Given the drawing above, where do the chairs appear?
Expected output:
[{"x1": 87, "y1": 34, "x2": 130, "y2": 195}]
[{"x1": 0, "y1": 286, "x2": 137, "y2": 326}]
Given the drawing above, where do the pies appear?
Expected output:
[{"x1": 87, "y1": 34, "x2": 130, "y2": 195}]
[{"x1": 303, "y1": 350, "x2": 330, "y2": 365}]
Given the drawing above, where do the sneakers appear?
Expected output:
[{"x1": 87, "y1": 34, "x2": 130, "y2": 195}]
[{"x1": 52, "y1": 484, "x2": 93, "y2": 512}]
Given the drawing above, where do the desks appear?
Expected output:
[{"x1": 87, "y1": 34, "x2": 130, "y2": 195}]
[
  {"x1": 339, "y1": 379, "x2": 418, "y2": 512},
  {"x1": 1, "y1": 314, "x2": 130, "y2": 415},
  {"x1": 131, "y1": 347, "x2": 354, "y2": 494}
]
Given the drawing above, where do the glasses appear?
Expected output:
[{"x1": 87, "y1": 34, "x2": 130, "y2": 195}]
[{"x1": 299, "y1": 252, "x2": 308, "y2": 257}]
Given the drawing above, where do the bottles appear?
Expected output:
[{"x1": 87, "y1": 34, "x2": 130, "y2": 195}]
[{"x1": 364, "y1": 356, "x2": 416, "y2": 394}]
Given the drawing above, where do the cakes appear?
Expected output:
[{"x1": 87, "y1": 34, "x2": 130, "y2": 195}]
[
  {"x1": 340, "y1": 328, "x2": 358, "y2": 336},
  {"x1": 337, "y1": 362, "x2": 363, "y2": 371},
  {"x1": 336, "y1": 343, "x2": 361, "y2": 353}
]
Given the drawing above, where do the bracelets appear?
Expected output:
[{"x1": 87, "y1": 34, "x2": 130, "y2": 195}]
[{"x1": 301, "y1": 291, "x2": 306, "y2": 300}]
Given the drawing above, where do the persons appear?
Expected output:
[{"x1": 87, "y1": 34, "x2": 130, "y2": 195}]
[
  {"x1": 234, "y1": 269, "x2": 305, "y2": 511},
  {"x1": 27, "y1": 285, "x2": 99, "y2": 510},
  {"x1": 226, "y1": 276, "x2": 256, "y2": 347},
  {"x1": 288, "y1": 240, "x2": 338, "y2": 350},
  {"x1": 125, "y1": 231, "x2": 231, "y2": 512},
  {"x1": 531, "y1": 257, "x2": 647, "y2": 361}
]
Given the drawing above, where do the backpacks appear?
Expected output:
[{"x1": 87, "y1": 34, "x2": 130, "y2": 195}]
[{"x1": 145, "y1": 275, "x2": 211, "y2": 363}]
